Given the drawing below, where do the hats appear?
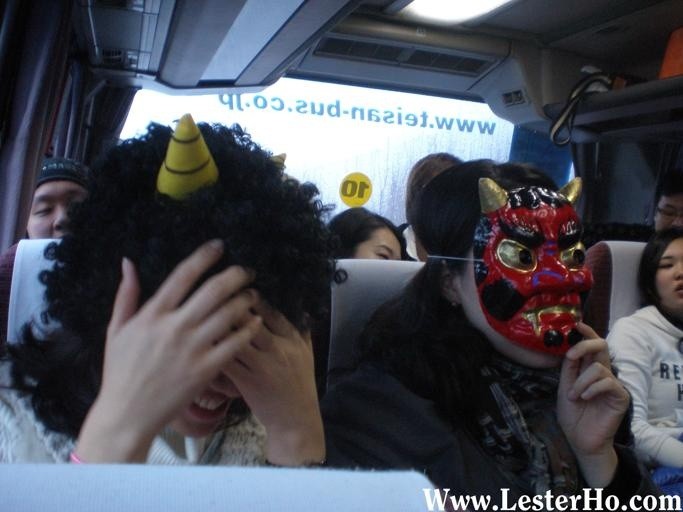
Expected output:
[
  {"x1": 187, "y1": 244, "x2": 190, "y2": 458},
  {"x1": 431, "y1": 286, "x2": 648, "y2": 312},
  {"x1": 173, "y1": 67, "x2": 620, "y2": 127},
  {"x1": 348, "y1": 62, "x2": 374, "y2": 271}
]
[{"x1": 32, "y1": 156, "x2": 96, "y2": 196}]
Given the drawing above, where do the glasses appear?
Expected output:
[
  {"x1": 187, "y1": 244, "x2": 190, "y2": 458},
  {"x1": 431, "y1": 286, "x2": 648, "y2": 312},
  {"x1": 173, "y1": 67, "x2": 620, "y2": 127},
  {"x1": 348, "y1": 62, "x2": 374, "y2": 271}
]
[{"x1": 656, "y1": 205, "x2": 683, "y2": 217}]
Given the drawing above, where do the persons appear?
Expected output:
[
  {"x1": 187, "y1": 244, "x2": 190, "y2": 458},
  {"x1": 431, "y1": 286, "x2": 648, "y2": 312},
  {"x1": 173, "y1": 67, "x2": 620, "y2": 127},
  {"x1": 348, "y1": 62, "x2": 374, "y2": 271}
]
[
  {"x1": 313, "y1": 159, "x2": 671, "y2": 510},
  {"x1": 321, "y1": 205, "x2": 407, "y2": 261},
  {"x1": 585, "y1": 171, "x2": 683, "y2": 244},
  {"x1": 0, "y1": 156, "x2": 93, "y2": 345},
  {"x1": 0, "y1": 113, "x2": 355, "y2": 471},
  {"x1": 601, "y1": 227, "x2": 683, "y2": 510},
  {"x1": 398, "y1": 149, "x2": 466, "y2": 263}
]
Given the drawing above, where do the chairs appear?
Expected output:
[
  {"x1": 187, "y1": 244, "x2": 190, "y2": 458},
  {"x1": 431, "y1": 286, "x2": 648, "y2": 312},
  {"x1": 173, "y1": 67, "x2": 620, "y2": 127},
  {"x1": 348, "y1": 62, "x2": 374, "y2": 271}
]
[{"x1": 1, "y1": 239, "x2": 648, "y2": 420}]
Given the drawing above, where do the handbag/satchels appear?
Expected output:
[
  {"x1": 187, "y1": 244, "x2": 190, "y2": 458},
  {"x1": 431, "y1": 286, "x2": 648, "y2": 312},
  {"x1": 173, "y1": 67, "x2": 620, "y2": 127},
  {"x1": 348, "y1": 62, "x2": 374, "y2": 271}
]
[{"x1": 547, "y1": 72, "x2": 649, "y2": 148}]
